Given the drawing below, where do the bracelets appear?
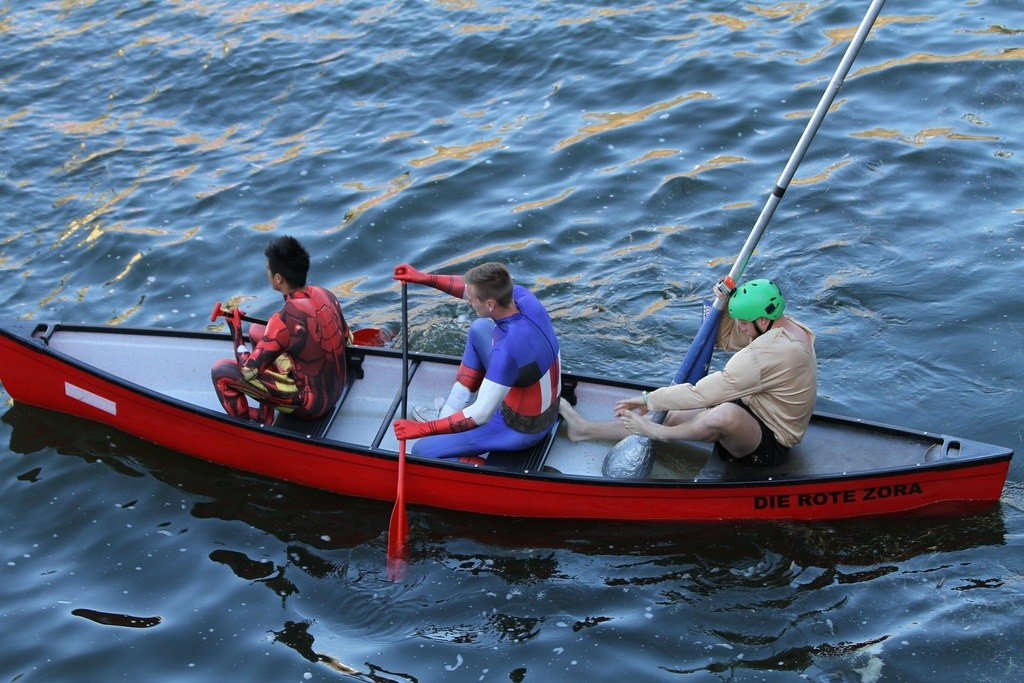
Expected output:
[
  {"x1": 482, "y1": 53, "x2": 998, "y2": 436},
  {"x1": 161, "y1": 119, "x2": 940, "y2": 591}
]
[{"x1": 641, "y1": 390, "x2": 648, "y2": 405}]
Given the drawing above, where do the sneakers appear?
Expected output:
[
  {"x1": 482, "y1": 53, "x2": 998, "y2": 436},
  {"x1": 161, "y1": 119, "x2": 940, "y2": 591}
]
[{"x1": 412, "y1": 398, "x2": 446, "y2": 423}]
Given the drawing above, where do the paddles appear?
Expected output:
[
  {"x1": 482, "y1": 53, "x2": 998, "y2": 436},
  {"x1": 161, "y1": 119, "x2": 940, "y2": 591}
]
[
  {"x1": 211, "y1": 302, "x2": 382, "y2": 348},
  {"x1": 387, "y1": 268, "x2": 408, "y2": 557}
]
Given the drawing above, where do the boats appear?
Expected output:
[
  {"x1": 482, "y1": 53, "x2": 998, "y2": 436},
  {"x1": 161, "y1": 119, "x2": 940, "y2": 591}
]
[{"x1": 1, "y1": 318, "x2": 1013, "y2": 522}]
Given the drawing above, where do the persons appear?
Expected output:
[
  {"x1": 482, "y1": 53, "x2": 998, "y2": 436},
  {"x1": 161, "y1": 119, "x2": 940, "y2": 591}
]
[
  {"x1": 210, "y1": 238, "x2": 348, "y2": 430},
  {"x1": 559, "y1": 278, "x2": 818, "y2": 470},
  {"x1": 392, "y1": 263, "x2": 562, "y2": 471}
]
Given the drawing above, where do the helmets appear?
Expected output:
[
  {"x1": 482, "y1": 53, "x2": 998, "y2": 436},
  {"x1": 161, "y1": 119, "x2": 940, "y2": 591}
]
[{"x1": 728, "y1": 279, "x2": 785, "y2": 322}]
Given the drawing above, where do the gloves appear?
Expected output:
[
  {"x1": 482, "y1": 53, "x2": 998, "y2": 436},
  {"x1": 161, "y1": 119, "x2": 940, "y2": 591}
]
[{"x1": 714, "y1": 275, "x2": 737, "y2": 301}]
